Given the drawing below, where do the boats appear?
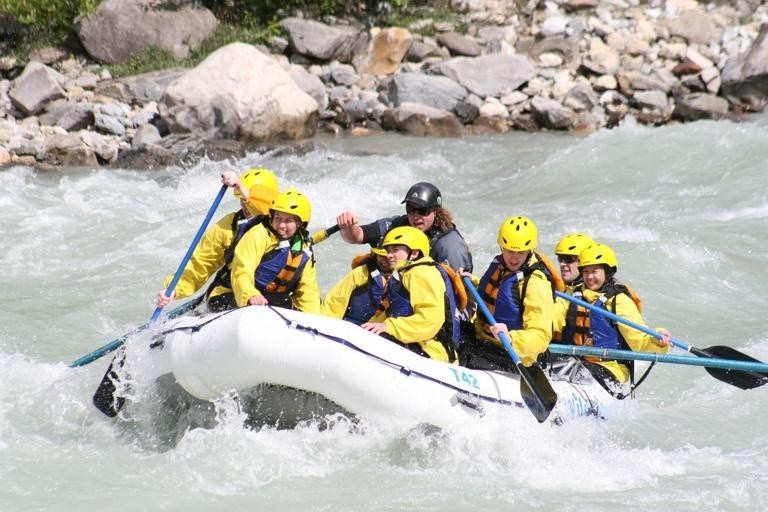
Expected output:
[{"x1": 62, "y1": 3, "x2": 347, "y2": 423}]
[{"x1": 129, "y1": 304, "x2": 637, "y2": 445}]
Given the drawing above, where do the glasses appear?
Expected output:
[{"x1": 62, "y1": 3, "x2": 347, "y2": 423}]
[
  {"x1": 406, "y1": 203, "x2": 437, "y2": 216},
  {"x1": 558, "y1": 254, "x2": 578, "y2": 263}
]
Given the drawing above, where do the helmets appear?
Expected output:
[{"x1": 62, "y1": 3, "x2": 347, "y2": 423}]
[
  {"x1": 268, "y1": 185, "x2": 312, "y2": 228},
  {"x1": 400, "y1": 182, "x2": 442, "y2": 209},
  {"x1": 370, "y1": 246, "x2": 388, "y2": 257},
  {"x1": 496, "y1": 215, "x2": 538, "y2": 252},
  {"x1": 576, "y1": 242, "x2": 618, "y2": 273},
  {"x1": 554, "y1": 233, "x2": 595, "y2": 257},
  {"x1": 233, "y1": 168, "x2": 280, "y2": 202},
  {"x1": 382, "y1": 225, "x2": 431, "y2": 258}
]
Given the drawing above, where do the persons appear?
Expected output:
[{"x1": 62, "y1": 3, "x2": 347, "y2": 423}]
[
  {"x1": 457, "y1": 217, "x2": 554, "y2": 373},
  {"x1": 337, "y1": 182, "x2": 474, "y2": 273},
  {"x1": 554, "y1": 233, "x2": 595, "y2": 341},
  {"x1": 155, "y1": 169, "x2": 278, "y2": 311},
  {"x1": 554, "y1": 244, "x2": 671, "y2": 389},
  {"x1": 323, "y1": 244, "x2": 389, "y2": 331},
  {"x1": 360, "y1": 226, "x2": 460, "y2": 366},
  {"x1": 208, "y1": 189, "x2": 320, "y2": 314}
]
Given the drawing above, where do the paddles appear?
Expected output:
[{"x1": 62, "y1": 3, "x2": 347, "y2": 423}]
[
  {"x1": 550, "y1": 283, "x2": 767, "y2": 391},
  {"x1": 457, "y1": 264, "x2": 560, "y2": 424},
  {"x1": 89, "y1": 176, "x2": 236, "y2": 418}
]
